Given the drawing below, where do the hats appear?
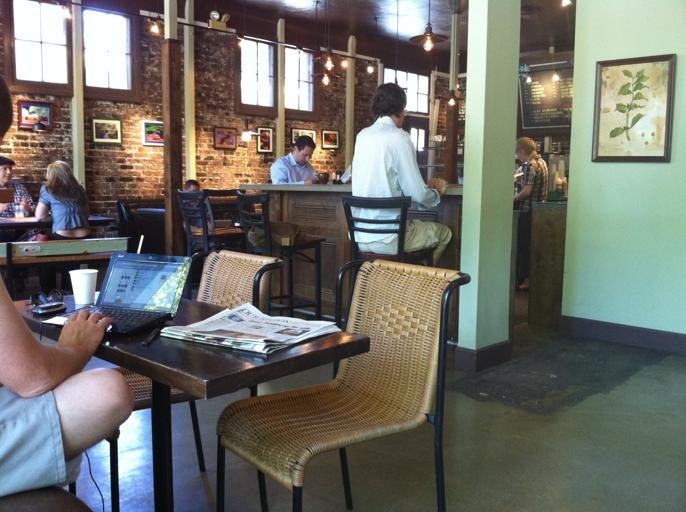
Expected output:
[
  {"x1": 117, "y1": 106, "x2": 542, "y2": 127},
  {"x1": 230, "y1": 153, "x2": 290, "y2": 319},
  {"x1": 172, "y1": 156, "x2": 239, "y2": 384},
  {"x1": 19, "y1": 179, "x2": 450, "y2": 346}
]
[{"x1": 0, "y1": 156, "x2": 15, "y2": 165}]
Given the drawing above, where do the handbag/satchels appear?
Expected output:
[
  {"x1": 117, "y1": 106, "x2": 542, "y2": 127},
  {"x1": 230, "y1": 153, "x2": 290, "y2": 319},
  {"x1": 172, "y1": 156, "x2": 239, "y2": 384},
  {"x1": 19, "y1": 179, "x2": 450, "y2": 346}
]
[
  {"x1": 247, "y1": 220, "x2": 302, "y2": 249},
  {"x1": 18, "y1": 228, "x2": 51, "y2": 243}
]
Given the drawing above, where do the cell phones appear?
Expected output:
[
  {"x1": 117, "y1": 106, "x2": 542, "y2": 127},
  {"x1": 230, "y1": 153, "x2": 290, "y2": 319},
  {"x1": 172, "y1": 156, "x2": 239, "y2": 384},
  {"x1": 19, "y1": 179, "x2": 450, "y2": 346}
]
[{"x1": 32, "y1": 302, "x2": 66, "y2": 315}]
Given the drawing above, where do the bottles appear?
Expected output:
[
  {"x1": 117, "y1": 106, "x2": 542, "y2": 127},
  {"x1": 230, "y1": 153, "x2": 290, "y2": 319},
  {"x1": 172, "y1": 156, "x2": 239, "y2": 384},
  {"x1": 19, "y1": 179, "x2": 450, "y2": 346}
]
[{"x1": 311, "y1": 170, "x2": 319, "y2": 183}]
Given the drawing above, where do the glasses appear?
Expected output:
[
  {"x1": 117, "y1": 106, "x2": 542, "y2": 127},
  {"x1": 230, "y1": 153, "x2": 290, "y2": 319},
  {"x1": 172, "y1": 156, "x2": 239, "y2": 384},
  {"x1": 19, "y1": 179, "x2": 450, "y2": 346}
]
[{"x1": 24, "y1": 289, "x2": 64, "y2": 308}]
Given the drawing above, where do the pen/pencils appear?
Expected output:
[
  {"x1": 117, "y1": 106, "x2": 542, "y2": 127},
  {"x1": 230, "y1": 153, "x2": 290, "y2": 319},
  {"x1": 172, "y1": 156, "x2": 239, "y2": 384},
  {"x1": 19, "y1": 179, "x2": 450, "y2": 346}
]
[{"x1": 142, "y1": 327, "x2": 160, "y2": 347}]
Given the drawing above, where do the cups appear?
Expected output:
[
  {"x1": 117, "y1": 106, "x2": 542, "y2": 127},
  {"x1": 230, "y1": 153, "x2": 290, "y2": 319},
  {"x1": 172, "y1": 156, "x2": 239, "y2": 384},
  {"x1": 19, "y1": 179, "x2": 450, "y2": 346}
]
[
  {"x1": 550, "y1": 158, "x2": 568, "y2": 200},
  {"x1": 13, "y1": 203, "x2": 25, "y2": 221},
  {"x1": 68, "y1": 268, "x2": 98, "y2": 305},
  {"x1": 317, "y1": 172, "x2": 329, "y2": 184}
]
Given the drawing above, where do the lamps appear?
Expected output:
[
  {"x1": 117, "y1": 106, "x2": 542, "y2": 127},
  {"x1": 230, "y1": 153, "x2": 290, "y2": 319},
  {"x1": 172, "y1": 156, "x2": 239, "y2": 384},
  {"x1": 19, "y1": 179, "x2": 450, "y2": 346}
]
[
  {"x1": 313, "y1": 0, "x2": 458, "y2": 105},
  {"x1": 208, "y1": 11, "x2": 230, "y2": 31}
]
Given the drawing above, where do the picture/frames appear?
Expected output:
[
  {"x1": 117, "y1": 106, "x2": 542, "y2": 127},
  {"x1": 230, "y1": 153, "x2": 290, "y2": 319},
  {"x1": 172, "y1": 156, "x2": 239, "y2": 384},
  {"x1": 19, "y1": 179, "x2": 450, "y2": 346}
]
[
  {"x1": 321, "y1": 129, "x2": 340, "y2": 150},
  {"x1": 256, "y1": 127, "x2": 274, "y2": 153},
  {"x1": 591, "y1": 54, "x2": 676, "y2": 162},
  {"x1": 17, "y1": 100, "x2": 55, "y2": 131},
  {"x1": 290, "y1": 127, "x2": 317, "y2": 146},
  {"x1": 141, "y1": 120, "x2": 165, "y2": 147},
  {"x1": 213, "y1": 127, "x2": 237, "y2": 150},
  {"x1": 90, "y1": 114, "x2": 123, "y2": 146}
]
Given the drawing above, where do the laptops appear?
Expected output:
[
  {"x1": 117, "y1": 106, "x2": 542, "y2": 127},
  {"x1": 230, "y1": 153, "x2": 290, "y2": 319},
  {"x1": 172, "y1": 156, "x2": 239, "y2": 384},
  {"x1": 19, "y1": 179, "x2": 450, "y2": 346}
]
[{"x1": 55, "y1": 252, "x2": 192, "y2": 334}]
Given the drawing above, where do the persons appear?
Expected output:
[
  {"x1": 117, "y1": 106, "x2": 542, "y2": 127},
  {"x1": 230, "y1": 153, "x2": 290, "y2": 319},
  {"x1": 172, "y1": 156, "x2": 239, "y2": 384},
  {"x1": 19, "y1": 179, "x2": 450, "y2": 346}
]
[
  {"x1": 270, "y1": 135, "x2": 318, "y2": 186},
  {"x1": 182, "y1": 180, "x2": 213, "y2": 236},
  {"x1": 350, "y1": 82, "x2": 453, "y2": 267},
  {"x1": 1, "y1": 74, "x2": 133, "y2": 497},
  {"x1": 514, "y1": 136, "x2": 548, "y2": 292},
  {"x1": 1, "y1": 157, "x2": 36, "y2": 218},
  {"x1": 35, "y1": 160, "x2": 92, "y2": 240}
]
